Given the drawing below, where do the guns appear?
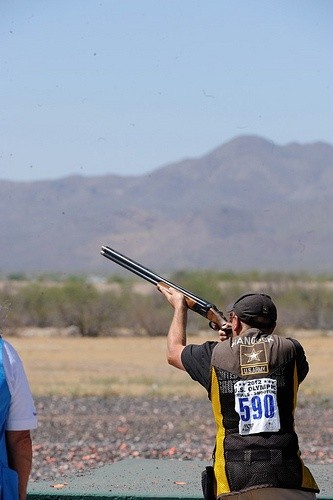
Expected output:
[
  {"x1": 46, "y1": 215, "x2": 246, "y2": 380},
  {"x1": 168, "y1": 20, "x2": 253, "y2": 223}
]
[{"x1": 98, "y1": 245, "x2": 234, "y2": 339}]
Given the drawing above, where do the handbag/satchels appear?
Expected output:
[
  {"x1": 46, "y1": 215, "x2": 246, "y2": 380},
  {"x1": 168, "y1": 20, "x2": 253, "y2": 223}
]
[{"x1": 201, "y1": 466, "x2": 216, "y2": 500}]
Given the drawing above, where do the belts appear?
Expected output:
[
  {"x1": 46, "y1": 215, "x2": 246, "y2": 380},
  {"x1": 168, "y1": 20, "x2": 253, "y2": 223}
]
[{"x1": 223, "y1": 448, "x2": 295, "y2": 462}]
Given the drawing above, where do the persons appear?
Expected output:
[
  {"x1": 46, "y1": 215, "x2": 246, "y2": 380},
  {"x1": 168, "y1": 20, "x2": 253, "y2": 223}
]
[
  {"x1": 153, "y1": 282, "x2": 320, "y2": 500},
  {"x1": 1, "y1": 335, "x2": 35, "y2": 500}
]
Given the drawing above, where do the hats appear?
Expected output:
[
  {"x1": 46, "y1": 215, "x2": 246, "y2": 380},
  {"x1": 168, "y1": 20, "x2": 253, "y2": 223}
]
[{"x1": 226, "y1": 292, "x2": 278, "y2": 328}]
